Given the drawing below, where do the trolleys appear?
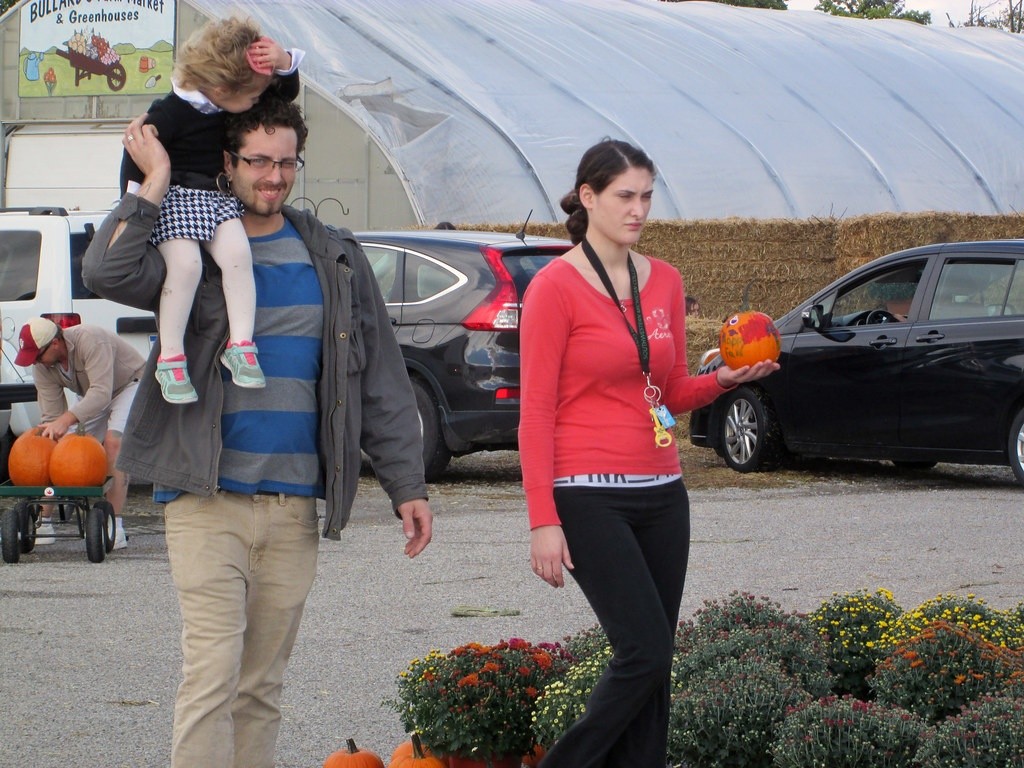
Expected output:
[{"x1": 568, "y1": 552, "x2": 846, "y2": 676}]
[{"x1": 0, "y1": 475, "x2": 116, "y2": 563}]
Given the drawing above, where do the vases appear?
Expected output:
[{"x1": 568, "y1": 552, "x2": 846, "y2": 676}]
[{"x1": 450, "y1": 755, "x2": 523, "y2": 768}]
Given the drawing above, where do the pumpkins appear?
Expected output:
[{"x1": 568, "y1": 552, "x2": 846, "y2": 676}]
[
  {"x1": 8, "y1": 423, "x2": 108, "y2": 488},
  {"x1": 719, "y1": 281, "x2": 781, "y2": 372},
  {"x1": 323, "y1": 731, "x2": 448, "y2": 768}
]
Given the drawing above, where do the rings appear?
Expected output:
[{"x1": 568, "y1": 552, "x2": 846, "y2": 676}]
[{"x1": 128, "y1": 135, "x2": 134, "y2": 140}]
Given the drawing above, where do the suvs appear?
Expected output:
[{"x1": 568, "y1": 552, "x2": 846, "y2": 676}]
[{"x1": 0, "y1": 207, "x2": 574, "y2": 483}]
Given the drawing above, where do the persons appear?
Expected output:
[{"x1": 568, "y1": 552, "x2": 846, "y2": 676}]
[
  {"x1": 14, "y1": 317, "x2": 147, "y2": 550},
  {"x1": 119, "y1": 17, "x2": 306, "y2": 404},
  {"x1": 435, "y1": 222, "x2": 456, "y2": 230},
  {"x1": 518, "y1": 134, "x2": 781, "y2": 768},
  {"x1": 80, "y1": 98, "x2": 434, "y2": 768}
]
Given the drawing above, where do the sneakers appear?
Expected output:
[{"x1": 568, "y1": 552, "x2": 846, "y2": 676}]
[
  {"x1": 219, "y1": 339, "x2": 266, "y2": 389},
  {"x1": 155, "y1": 353, "x2": 198, "y2": 405}
]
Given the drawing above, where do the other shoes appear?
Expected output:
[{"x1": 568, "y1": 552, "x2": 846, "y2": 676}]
[
  {"x1": 103, "y1": 526, "x2": 127, "y2": 550},
  {"x1": 17, "y1": 523, "x2": 55, "y2": 545}
]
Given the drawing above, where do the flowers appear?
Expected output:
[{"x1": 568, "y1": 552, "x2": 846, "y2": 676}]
[{"x1": 391, "y1": 637, "x2": 576, "y2": 758}]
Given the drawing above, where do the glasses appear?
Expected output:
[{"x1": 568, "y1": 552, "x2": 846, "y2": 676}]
[
  {"x1": 35, "y1": 342, "x2": 53, "y2": 363},
  {"x1": 229, "y1": 150, "x2": 304, "y2": 174}
]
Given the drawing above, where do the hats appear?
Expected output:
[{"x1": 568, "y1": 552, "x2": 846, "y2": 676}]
[{"x1": 14, "y1": 317, "x2": 58, "y2": 366}]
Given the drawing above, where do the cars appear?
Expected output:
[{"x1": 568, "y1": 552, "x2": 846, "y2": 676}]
[{"x1": 689, "y1": 238, "x2": 1024, "y2": 487}]
